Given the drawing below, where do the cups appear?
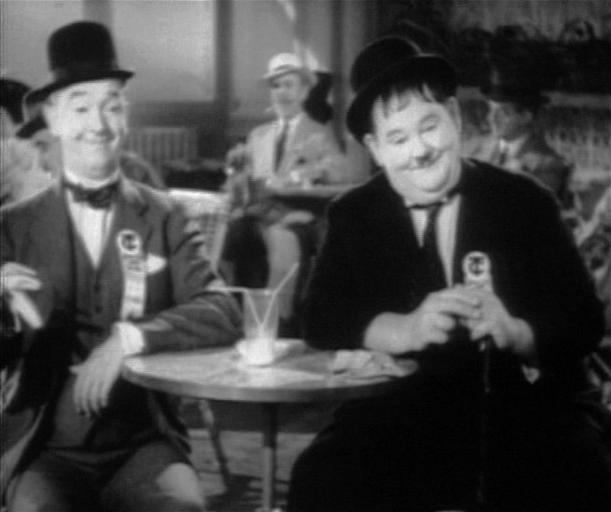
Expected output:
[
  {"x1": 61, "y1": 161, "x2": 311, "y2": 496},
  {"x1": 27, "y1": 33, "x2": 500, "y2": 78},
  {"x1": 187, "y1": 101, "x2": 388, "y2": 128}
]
[{"x1": 242, "y1": 288, "x2": 278, "y2": 366}]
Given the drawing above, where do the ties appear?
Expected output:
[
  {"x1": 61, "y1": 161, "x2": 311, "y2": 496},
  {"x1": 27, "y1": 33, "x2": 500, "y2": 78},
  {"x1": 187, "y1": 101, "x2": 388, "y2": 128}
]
[
  {"x1": 275, "y1": 124, "x2": 289, "y2": 168},
  {"x1": 410, "y1": 202, "x2": 479, "y2": 371}
]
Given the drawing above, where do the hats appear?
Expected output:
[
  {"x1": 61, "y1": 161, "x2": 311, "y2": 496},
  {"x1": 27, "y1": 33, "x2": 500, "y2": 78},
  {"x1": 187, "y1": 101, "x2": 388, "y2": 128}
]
[
  {"x1": 261, "y1": 53, "x2": 320, "y2": 90},
  {"x1": 481, "y1": 56, "x2": 553, "y2": 104},
  {"x1": 346, "y1": 35, "x2": 460, "y2": 148},
  {"x1": 1, "y1": 20, "x2": 135, "y2": 138}
]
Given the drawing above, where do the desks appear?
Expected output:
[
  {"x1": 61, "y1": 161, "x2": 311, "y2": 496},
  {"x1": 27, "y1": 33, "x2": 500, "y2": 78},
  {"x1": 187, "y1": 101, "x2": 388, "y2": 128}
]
[{"x1": 118, "y1": 341, "x2": 418, "y2": 512}]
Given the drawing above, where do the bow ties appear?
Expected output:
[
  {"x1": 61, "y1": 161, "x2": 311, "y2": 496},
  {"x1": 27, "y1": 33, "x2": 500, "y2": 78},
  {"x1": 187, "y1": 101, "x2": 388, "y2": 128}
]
[{"x1": 72, "y1": 181, "x2": 119, "y2": 208}]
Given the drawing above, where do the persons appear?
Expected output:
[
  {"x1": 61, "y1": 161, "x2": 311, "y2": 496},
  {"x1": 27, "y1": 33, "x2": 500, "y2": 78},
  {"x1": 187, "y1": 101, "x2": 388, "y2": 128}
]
[
  {"x1": 12, "y1": 89, "x2": 170, "y2": 190},
  {"x1": 0, "y1": 19, "x2": 245, "y2": 511},
  {"x1": 0, "y1": 79, "x2": 38, "y2": 207},
  {"x1": 284, "y1": 33, "x2": 611, "y2": 511},
  {"x1": 230, "y1": 53, "x2": 335, "y2": 198},
  {"x1": 477, "y1": 64, "x2": 574, "y2": 212}
]
